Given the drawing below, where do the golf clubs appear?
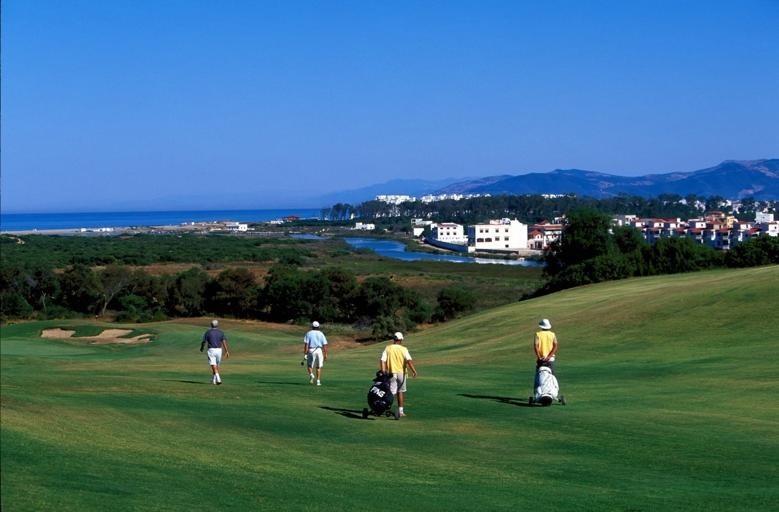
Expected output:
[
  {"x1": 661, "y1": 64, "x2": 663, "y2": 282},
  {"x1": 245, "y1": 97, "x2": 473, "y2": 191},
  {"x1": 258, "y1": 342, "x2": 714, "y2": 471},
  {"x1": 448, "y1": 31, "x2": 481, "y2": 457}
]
[{"x1": 301, "y1": 345, "x2": 319, "y2": 365}]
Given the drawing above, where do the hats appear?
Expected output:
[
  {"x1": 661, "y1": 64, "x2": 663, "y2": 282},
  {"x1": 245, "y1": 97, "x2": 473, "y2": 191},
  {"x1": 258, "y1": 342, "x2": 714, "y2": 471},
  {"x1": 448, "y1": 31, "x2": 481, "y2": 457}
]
[
  {"x1": 392, "y1": 331, "x2": 405, "y2": 341},
  {"x1": 312, "y1": 320, "x2": 320, "y2": 328},
  {"x1": 210, "y1": 319, "x2": 219, "y2": 327},
  {"x1": 538, "y1": 317, "x2": 552, "y2": 330}
]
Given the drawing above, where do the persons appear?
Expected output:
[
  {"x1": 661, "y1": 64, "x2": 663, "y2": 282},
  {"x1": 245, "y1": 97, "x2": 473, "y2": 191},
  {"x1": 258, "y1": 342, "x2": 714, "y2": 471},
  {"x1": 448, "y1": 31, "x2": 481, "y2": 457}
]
[
  {"x1": 303, "y1": 319, "x2": 329, "y2": 387},
  {"x1": 533, "y1": 319, "x2": 559, "y2": 396},
  {"x1": 379, "y1": 331, "x2": 418, "y2": 416},
  {"x1": 200, "y1": 318, "x2": 232, "y2": 386}
]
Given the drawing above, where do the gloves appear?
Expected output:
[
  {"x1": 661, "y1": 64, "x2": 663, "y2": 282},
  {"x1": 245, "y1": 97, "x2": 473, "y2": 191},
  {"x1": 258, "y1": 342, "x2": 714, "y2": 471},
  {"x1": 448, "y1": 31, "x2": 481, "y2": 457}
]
[
  {"x1": 303, "y1": 354, "x2": 308, "y2": 361},
  {"x1": 199, "y1": 342, "x2": 206, "y2": 353}
]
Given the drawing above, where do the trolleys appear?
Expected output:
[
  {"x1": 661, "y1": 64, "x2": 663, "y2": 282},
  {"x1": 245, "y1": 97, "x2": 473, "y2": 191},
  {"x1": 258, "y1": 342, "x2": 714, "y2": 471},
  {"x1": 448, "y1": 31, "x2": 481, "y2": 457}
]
[
  {"x1": 529, "y1": 360, "x2": 565, "y2": 408},
  {"x1": 362, "y1": 370, "x2": 400, "y2": 419}
]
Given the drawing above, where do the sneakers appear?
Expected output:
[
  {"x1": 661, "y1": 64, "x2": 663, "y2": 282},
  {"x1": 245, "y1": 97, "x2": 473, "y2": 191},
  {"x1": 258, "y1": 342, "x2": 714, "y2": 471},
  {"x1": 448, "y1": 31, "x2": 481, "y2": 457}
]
[
  {"x1": 211, "y1": 380, "x2": 223, "y2": 386},
  {"x1": 308, "y1": 375, "x2": 323, "y2": 386},
  {"x1": 397, "y1": 410, "x2": 408, "y2": 419}
]
